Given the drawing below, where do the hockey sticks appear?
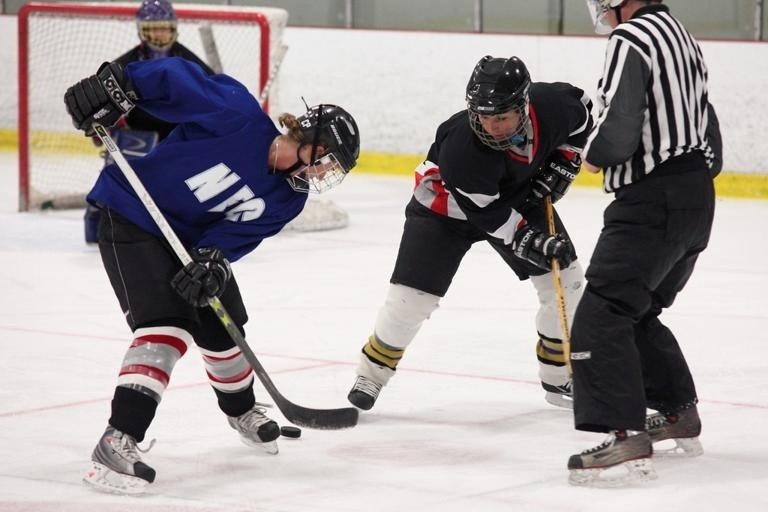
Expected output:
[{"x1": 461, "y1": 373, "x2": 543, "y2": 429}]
[{"x1": 93, "y1": 121, "x2": 358, "y2": 430}]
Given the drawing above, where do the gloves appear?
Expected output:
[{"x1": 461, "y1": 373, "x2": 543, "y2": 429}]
[
  {"x1": 171, "y1": 246, "x2": 232, "y2": 309},
  {"x1": 63, "y1": 61, "x2": 138, "y2": 136},
  {"x1": 527, "y1": 150, "x2": 582, "y2": 207},
  {"x1": 510, "y1": 225, "x2": 573, "y2": 271}
]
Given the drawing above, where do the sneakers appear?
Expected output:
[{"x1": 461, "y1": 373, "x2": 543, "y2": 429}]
[
  {"x1": 348, "y1": 374, "x2": 383, "y2": 410},
  {"x1": 567, "y1": 429, "x2": 651, "y2": 469},
  {"x1": 227, "y1": 402, "x2": 281, "y2": 442},
  {"x1": 92, "y1": 424, "x2": 156, "y2": 483},
  {"x1": 542, "y1": 378, "x2": 574, "y2": 395},
  {"x1": 644, "y1": 407, "x2": 701, "y2": 440}
]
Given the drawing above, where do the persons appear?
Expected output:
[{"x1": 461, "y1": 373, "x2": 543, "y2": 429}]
[
  {"x1": 64, "y1": 56, "x2": 360, "y2": 483},
  {"x1": 111, "y1": 0, "x2": 215, "y2": 76},
  {"x1": 348, "y1": 56, "x2": 594, "y2": 410},
  {"x1": 568, "y1": 0, "x2": 721, "y2": 470}
]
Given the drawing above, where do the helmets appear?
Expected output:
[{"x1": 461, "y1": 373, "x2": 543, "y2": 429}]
[
  {"x1": 288, "y1": 105, "x2": 360, "y2": 194},
  {"x1": 136, "y1": 1, "x2": 178, "y2": 53},
  {"x1": 465, "y1": 55, "x2": 532, "y2": 151}
]
